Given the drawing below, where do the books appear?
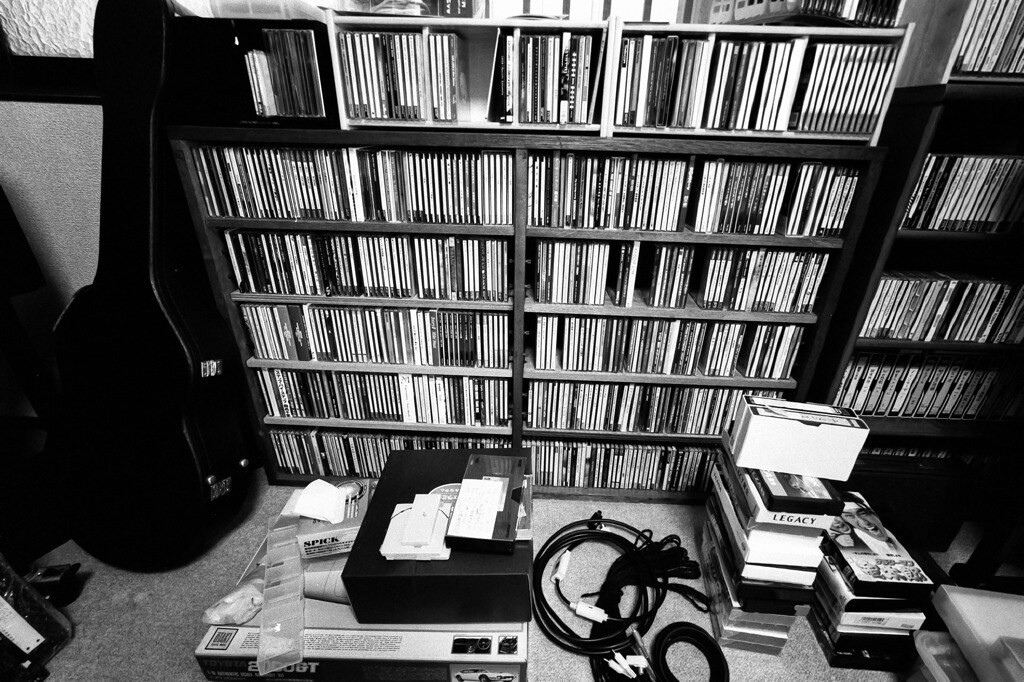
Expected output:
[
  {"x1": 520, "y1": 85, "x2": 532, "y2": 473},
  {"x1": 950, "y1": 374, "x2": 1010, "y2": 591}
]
[
  {"x1": 532, "y1": 235, "x2": 829, "y2": 313},
  {"x1": 205, "y1": 0, "x2": 904, "y2": 27},
  {"x1": 243, "y1": 29, "x2": 468, "y2": 121},
  {"x1": 860, "y1": 442, "x2": 989, "y2": 463},
  {"x1": 899, "y1": 152, "x2": 1024, "y2": 232},
  {"x1": 527, "y1": 379, "x2": 784, "y2": 437},
  {"x1": 526, "y1": 153, "x2": 862, "y2": 240},
  {"x1": 268, "y1": 429, "x2": 525, "y2": 561},
  {"x1": 696, "y1": 396, "x2": 935, "y2": 672},
  {"x1": 833, "y1": 352, "x2": 1022, "y2": 422},
  {"x1": 954, "y1": 0, "x2": 1024, "y2": 75},
  {"x1": 219, "y1": 230, "x2": 510, "y2": 302},
  {"x1": 521, "y1": 440, "x2": 716, "y2": 493},
  {"x1": 255, "y1": 371, "x2": 513, "y2": 428},
  {"x1": 858, "y1": 270, "x2": 1024, "y2": 344},
  {"x1": 191, "y1": 140, "x2": 514, "y2": 226},
  {"x1": 534, "y1": 316, "x2": 804, "y2": 381},
  {"x1": 498, "y1": 28, "x2": 900, "y2": 135},
  {"x1": 240, "y1": 304, "x2": 511, "y2": 370}
]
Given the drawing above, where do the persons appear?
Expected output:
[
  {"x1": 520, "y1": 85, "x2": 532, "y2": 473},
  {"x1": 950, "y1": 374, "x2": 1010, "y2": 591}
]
[
  {"x1": 833, "y1": 492, "x2": 888, "y2": 553},
  {"x1": 828, "y1": 516, "x2": 871, "y2": 551}
]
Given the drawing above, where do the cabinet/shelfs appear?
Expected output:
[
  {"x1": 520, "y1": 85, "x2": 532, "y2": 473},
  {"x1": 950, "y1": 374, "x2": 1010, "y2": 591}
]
[{"x1": 167, "y1": 0, "x2": 1023, "y2": 506}]
